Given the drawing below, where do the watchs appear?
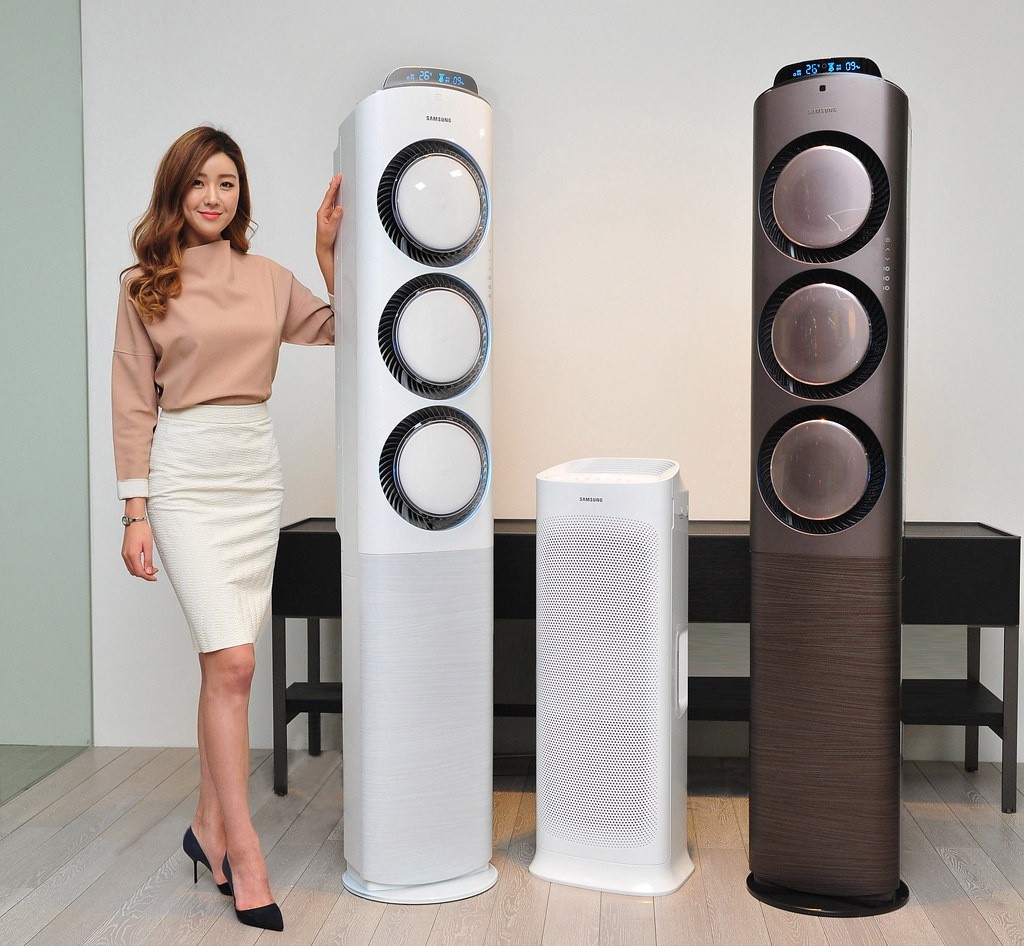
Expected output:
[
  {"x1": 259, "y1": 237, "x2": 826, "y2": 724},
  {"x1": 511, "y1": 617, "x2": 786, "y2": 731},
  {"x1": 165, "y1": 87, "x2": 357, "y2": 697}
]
[{"x1": 122, "y1": 516, "x2": 146, "y2": 526}]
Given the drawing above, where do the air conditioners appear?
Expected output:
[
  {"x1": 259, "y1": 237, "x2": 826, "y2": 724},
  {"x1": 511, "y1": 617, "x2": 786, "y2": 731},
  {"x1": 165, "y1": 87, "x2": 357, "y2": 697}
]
[
  {"x1": 334, "y1": 66, "x2": 499, "y2": 904},
  {"x1": 746, "y1": 57, "x2": 910, "y2": 918}
]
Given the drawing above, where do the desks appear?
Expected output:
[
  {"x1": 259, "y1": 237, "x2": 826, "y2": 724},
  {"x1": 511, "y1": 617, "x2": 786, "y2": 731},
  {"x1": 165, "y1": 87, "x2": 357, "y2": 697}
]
[{"x1": 271, "y1": 517, "x2": 1022, "y2": 814}]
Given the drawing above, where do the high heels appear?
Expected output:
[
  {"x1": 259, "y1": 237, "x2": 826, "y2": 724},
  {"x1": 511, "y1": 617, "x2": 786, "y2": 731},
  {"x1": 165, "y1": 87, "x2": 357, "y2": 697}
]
[
  {"x1": 222, "y1": 851, "x2": 291, "y2": 931},
  {"x1": 184, "y1": 826, "x2": 251, "y2": 896}
]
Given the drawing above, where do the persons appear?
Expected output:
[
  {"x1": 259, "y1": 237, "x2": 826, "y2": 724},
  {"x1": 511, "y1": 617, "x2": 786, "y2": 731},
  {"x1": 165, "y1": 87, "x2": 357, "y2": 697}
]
[{"x1": 111, "y1": 126, "x2": 343, "y2": 931}]
